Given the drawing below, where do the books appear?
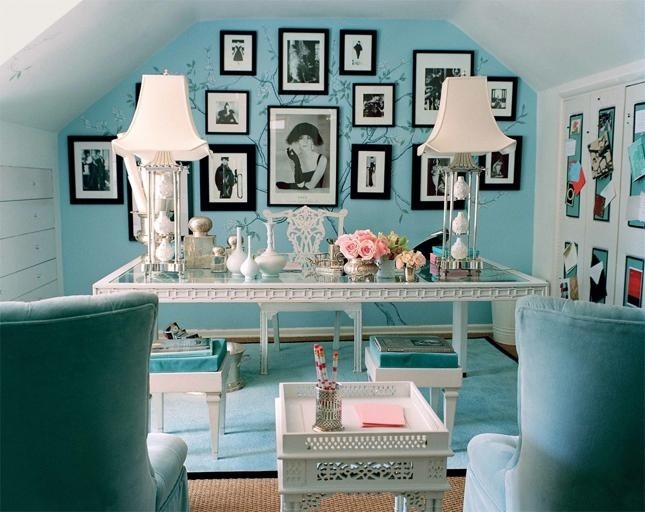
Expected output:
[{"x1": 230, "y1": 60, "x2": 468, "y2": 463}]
[
  {"x1": 149, "y1": 337, "x2": 213, "y2": 359},
  {"x1": 374, "y1": 336, "x2": 456, "y2": 353}
]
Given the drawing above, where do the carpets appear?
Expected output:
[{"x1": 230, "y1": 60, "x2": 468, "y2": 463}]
[{"x1": 146, "y1": 335, "x2": 518, "y2": 479}]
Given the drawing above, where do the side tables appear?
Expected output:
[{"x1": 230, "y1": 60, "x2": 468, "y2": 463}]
[{"x1": 273, "y1": 380, "x2": 453, "y2": 512}]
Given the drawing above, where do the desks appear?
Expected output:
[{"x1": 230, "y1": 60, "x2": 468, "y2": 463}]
[{"x1": 88, "y1": 248, "x2": 550, "y2": 377}]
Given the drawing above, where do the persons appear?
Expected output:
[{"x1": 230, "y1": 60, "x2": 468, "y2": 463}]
[
  {"x1": 275, "y1": 123, "x2": 327, "y2": 190},
  {"x1": 430, "y1": 159, "x2": 445, "y2": 196},
  {"x1": 215, "y1": 157, "x2": 237, "y2": 199},
  {"x1": 353, "y1": 40, "x2": 363, "y2": 58},
  {"x1": 231, "y1": 40, "x2": 244, "y2": 61},
  {"x1": 217, "y1": 103, "x2": 239, "y2": 124},
  {"x1": 430, "y1": 70, "x2": 442, "y2": 111},
  {"x1": 367, "y1": 156, "x2": 376, "y2": 188},
  {"x1": 363, "y1": 94, "x2": 384, "y2": 117},
  {"x1": 82, "y1": 150, "x2": 111, "y2": 191}
]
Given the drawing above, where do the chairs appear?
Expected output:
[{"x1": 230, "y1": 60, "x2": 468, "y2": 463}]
[{"x1": 259, "y1": 204, "x2": 364, "y2": 374}]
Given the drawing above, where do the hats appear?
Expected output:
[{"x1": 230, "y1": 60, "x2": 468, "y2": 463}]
[{"x1": 286, "y1": 123, "x2": 323, "y2": 146}]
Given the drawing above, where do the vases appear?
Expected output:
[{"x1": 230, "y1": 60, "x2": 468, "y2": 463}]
[
  {"x1": 343, "y1": 257, "x2": 379, "y2": 281},
  {"x1": 379, "y1": 260, "x2": 396, "y2": 280},
  {"x1": 405, "y1": 266, "x2": 417, "y2": 282}
]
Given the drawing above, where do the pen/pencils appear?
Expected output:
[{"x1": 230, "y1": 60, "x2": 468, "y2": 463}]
[{"x1": 313, "y1": 343, "x2": 339, "y2": 389}]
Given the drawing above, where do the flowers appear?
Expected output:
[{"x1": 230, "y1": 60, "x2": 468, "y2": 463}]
[
  {"x1": 375, "y1": 232, "x2": 408, "y2": 262},
  {"x1": 335, "y1": 229, "x2": 390, "y2": 261},
  {"x1": 392, "y1": 251, "x2": 427, "y2": 275}
]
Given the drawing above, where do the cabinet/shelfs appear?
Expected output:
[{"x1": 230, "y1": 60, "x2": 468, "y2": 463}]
[{"x1": 0, "y1": 159, "x2": 61, "y2": 302}]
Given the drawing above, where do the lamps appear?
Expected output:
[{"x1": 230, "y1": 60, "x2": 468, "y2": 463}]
[
  {"x1": 416, "y1": 73, "x2": 516, "y2": 268},
  {"x1": 111, "y1": 70, "x2": 212, "y2": 278}
]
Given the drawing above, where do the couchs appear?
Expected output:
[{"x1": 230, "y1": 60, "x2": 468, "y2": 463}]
[
  {"x1": 1, "y1": 289, "x2": 188, "y2": 512},
  {"x1": 464, "y1": 294, "x2": 645, "y2": 512}
]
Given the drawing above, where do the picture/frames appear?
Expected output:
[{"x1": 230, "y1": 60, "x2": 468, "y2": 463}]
[
  {"x1": 561, "y1": 241, "x2": 579, "y2": 300},
  {"x1": 279, "y1": 28, "x2": 329, "y2": 94},
  {"x1": 129, "y1": 161, "x2": 193, "y2": 244},
  {"x1": 589, "y1": 247, "x2": 608, "y2": 304},
  {"x1": 340, "y1": 29, "x2": 376, "y2": 75},
  {"x1": 593, "y1": 106, "x2": 616, "y2": 222},
  {"x1": 66, "y1": 136, "x2": 124, "y2": 205},
  {"x1": 479, "y1": 135, "x2": 523, "y2": 190},
  {"x1": 205, "y1": 90, "x2": 249, "y2": 134},
  {"x1": 623, "y1": 255, "x2": 645, "y2": 308},
  {"x1": 627, "y1": 103, "x2": 645, "y2": 229},
  {"x1": 267, "y1": 106, "x2": 338, "y2": 208},
  {"x1": 220, "y1": 32, "x2": 257, "y2": 75},
  {"x1": 485, "y1": 76, "x2": 517, "y2": 121},
  {"x1": 353, "y1": 83, "x2": 395, "y2": 126},
  {"x1": 350, "y1": 144, "x2": 392, "y2": 199},
  {"x1": 412, "y1": 143, "x2": 466, "y2": 210},
  {"x1": 201, "y1": 144, "x2": 258, "y2": 212},
  {"x1": 413, "y1": 50, "x2": 474, "y2": 127},
  {"x1": 566, "y1": 114, "x2": 583, "y2": 219}
]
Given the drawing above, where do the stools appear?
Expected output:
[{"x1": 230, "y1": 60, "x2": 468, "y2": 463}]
[
  {"x1": 366, "y1": 340, "x2": 460, "y2": 464},
  {"x1": 149, "y1": 336, "x2": 230, "y2": 466}
]
